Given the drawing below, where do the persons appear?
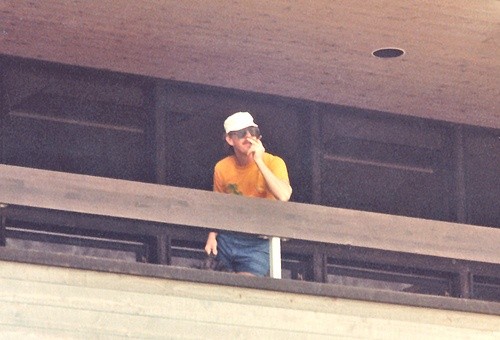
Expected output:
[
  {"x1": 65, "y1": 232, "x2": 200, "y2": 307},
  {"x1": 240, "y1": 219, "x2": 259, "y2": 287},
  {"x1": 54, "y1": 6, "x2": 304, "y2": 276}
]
[{"x1": 204, "y1": 111, "x2": 292, "y2": 277}]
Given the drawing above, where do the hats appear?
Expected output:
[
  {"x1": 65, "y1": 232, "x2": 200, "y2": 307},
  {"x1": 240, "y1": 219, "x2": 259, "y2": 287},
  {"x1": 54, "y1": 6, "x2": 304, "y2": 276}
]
[{"x1": 223, "y1": 111, "x2": 259, "y2": 134}]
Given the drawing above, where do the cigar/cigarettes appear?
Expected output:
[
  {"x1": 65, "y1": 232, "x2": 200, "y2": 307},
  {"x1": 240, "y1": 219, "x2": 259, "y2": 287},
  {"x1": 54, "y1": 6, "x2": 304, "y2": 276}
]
[{"x1": 249, "y1": 135, "x2": 263, "y2": 144}]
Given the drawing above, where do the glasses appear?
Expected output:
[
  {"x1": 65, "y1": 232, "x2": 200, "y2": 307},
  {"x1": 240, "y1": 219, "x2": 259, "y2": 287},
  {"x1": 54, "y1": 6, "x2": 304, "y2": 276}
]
[{"x1": 231, "y1": 127, "x2": 261, "y2": 139}]
